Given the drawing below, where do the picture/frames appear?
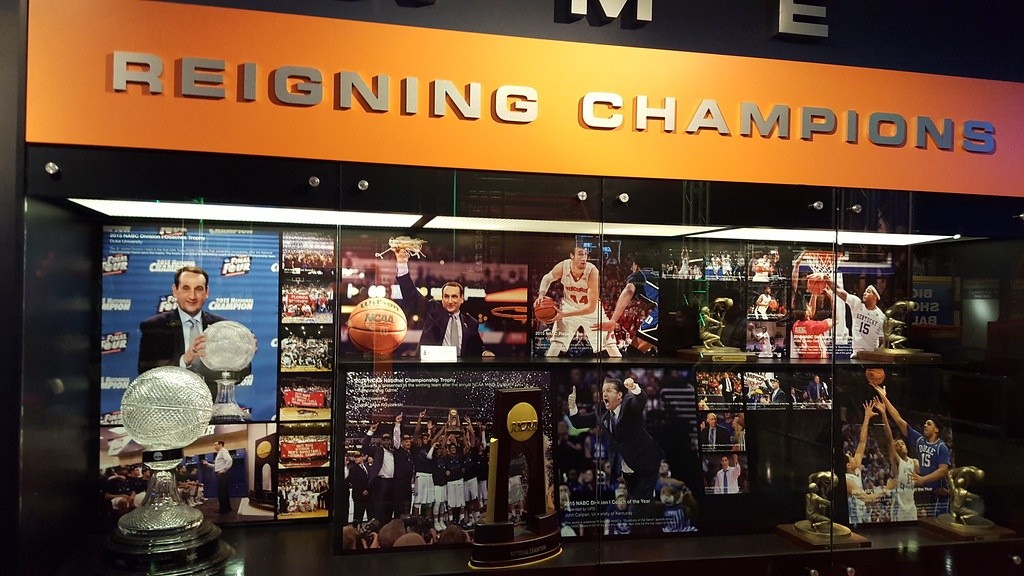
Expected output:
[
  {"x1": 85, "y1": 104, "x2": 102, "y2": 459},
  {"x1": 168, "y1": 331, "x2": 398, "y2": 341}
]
[{"x1": 912, "y1": 276, "x2": 961, "y2": 324}]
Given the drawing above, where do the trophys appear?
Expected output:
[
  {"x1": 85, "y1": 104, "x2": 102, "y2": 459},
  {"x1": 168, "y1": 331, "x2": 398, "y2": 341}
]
[
  {"x1": 850, "y1": 301, "x2": 944, "y2": 363},
  {"x1": 935, "y1": 466, "x2": 1012, "y2": 539},
  {"x1": 675, "y1": 298, "x2": 759, "y2": 362},
  {"x1": 780, "y1": 472, "x2": 872, "y2": 550},
  {"x1": 467, "y1": 387, "x2": 562, "y2": 569},
  {"x1": 199, "y1": 320, "x2": 255, "y2": 419},
  {"x1": 115, "y1": 366, "x2": 236, "y2": 576}
]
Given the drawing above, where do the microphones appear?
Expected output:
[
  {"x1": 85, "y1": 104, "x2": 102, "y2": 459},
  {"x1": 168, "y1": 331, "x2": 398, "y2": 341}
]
[{"x1": 427, "y1": 312, "x2": 449, "y2": 346}]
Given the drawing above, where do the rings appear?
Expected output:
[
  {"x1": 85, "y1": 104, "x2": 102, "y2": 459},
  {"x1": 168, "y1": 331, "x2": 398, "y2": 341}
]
[{"x1": 193, "y1": 346, "x2": 196, "y2": 352}]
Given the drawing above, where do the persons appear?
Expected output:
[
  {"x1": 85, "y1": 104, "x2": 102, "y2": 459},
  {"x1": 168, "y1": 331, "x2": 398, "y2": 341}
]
[
  {"x1": 282, "y1": 283, "x2": 333, "y2": 317},
  {"x1": 533, "y1": 247, "x2": 621, "y2": 357},
  {"x1": 664, "y1": 244, "x2": 953, "y2": 523},
  {"x1": 201, "y1": 441, "x2": 232, "y2": 513},
  {"x1": 100, "y1": 465, "x2": 208, "y2": 511},
  {"x1": 278, "y1": 477, "x2": 328, "y2": 516},
  {"x1": 590, "y1": 255, "x2": 660, "y2": 357},
  {"x1": 281, "y1": 334, "x2": 327, "y2": 369},
  {"x1": 343, "y1": 406, "x2": 491, "y2": 548},
  {"x1": 560, "y1": 366, "x2": 699, "y2": 536},
  {"x1": 285, "y1": 249, "x2": 335, "y2": 269},
  {"x1": 137, "y1": 266, "x2": 251, "y2": 402},
  {"x1": 396, "y1": 247, "x2": 495, "y2": 356}
]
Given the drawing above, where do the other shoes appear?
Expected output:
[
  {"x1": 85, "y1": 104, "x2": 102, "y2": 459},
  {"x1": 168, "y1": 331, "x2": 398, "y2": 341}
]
[
  {"x1": 431, "y1": 516, "x2": 478, "y2": 531},
  {"x1": 213, "y1": 510, "x2": 221, "y2": 513}
]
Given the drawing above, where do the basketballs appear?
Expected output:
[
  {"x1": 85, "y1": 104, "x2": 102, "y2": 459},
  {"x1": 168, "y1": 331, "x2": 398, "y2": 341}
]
[
  {"x1": 718, "y1": 261, "x2": 721, "y2": 265},
  {"x1": 755, "y1": 266, "x2": 762, "y2": 273},
  {"x1": 807, "y1": 276, "x2": 827, "y2": 295},
  {"x1": 534, "y1": 299, "x2": 558, "y2": 322},
  {"x1": 866, "y1": 368, "x2": 885, "y2": 384},
  {"x1": 347, "y1": 296, "x2": 408, "y2": 355},
  {"x1": 770, "y1": 300, "x2": 777, "y2": 309}
]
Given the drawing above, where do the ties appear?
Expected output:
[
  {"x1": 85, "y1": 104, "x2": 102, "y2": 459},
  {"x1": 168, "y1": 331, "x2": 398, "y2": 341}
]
[
  {"x1": 710, "y1": 429, "x2": 714, "y2": 443},
  {"x1": 611, "y1": 413, "x2": 621, "y2": 474},
  {"x1": 451, "y1": 315, "x2": 459, "y2": 356},
  {"x1": 723, "y1": 470, "x2": 728, "y2": 487},
  {"x1": 188, "y1": 319, "x2": 201, "y2": 349}
]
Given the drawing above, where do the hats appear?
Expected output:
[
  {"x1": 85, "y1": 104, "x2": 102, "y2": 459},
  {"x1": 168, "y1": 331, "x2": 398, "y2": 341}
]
[
  {"x1": 381, "y1": 434, "x2": 392, "y2": 439},
  {"x1": 421, "y1": 432, "x2": 429, "y2": 438},
  {"x1": 401, "y1": 435, "x2": 411, "y2": 441}
]
